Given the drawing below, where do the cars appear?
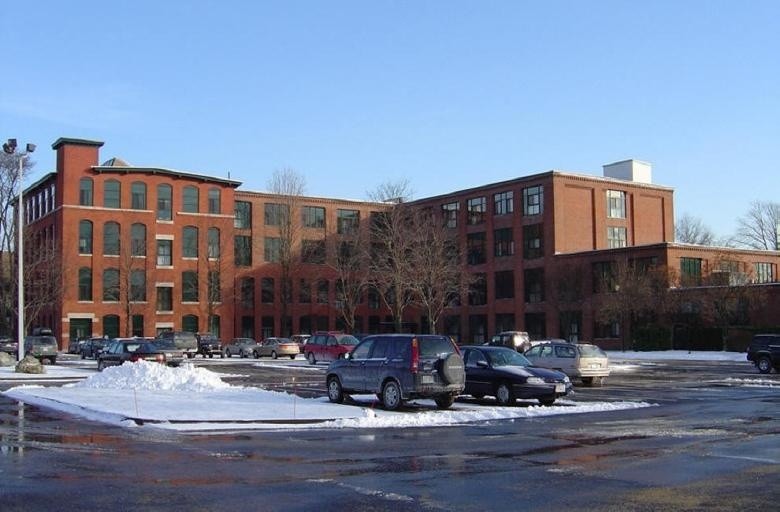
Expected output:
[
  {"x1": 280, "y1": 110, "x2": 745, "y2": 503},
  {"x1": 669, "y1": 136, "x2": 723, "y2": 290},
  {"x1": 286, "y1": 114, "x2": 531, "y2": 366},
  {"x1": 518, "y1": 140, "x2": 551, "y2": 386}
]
[
  {"x1": 188, "y1": 332, "x2": 310, "y2": 359},
  {"x1": 67, "y1": 329, "x2": 197, "y2": 372},
  {"x1": 460, "y1": 343, "x2": 609, "y2": 406},
  {"x1": 0, "y1": 336, "x2": 18, "y2": 353}
]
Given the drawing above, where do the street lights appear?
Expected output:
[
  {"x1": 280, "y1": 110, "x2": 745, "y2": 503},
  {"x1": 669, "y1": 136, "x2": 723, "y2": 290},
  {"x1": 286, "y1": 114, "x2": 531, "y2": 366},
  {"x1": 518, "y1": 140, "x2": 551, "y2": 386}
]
[{"x1": 3, "y1": 139, "x2": 35, "y2": 362}]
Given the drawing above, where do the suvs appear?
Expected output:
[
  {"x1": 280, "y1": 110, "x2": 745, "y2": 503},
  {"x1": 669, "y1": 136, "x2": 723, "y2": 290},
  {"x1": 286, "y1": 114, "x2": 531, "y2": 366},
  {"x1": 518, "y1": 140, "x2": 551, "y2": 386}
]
[
  {"x1": 304, "y1": 331, "x2": 359, "y2": 364},
  {"x1": 325, "y1": 333, "x2": 465, "y2": 409},
  {"x1": 746, "y1": 333, "x2": 780, "y2": 375},
  {"x1": 489, "y1": 330, "x2": 532, "y2": 354},
  {"x1": 24, "y1": 336, "x2": 58, "y2": 365}
]
